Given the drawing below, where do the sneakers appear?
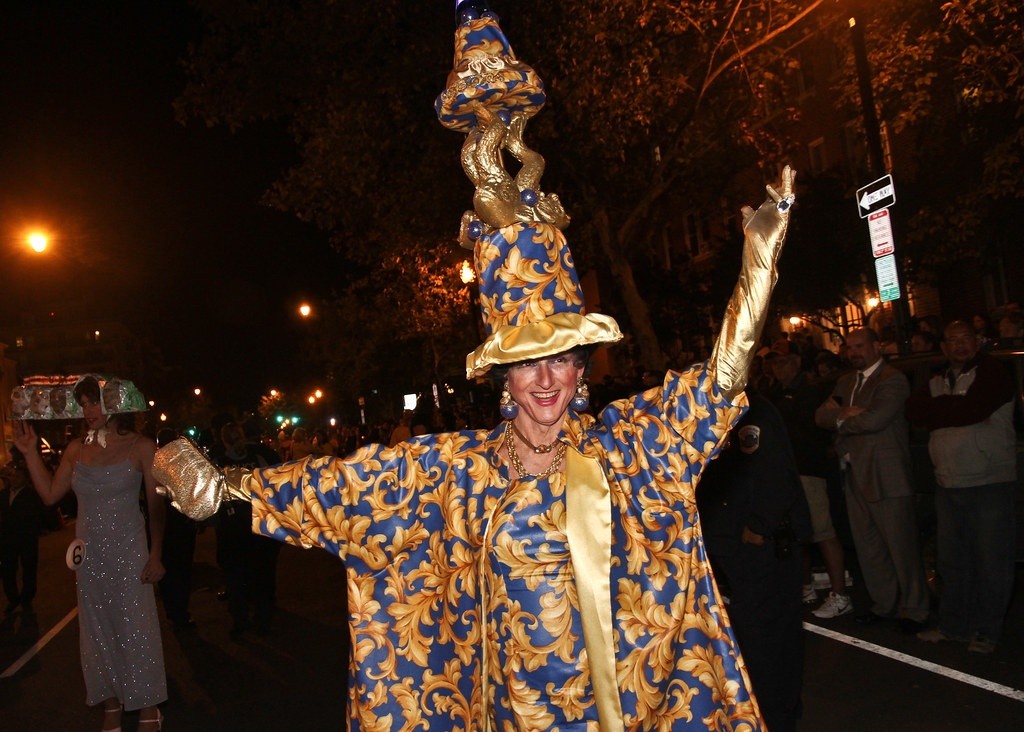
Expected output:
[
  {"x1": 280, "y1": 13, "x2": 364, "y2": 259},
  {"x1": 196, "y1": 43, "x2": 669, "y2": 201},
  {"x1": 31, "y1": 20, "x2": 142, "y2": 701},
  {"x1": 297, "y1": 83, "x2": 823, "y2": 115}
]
[
  {"x1": 802, "y1": 584, "x2": 816, "y2": 603},
  {"x1": 814, "y1": 591, "x2": 856, "y2": 618}
]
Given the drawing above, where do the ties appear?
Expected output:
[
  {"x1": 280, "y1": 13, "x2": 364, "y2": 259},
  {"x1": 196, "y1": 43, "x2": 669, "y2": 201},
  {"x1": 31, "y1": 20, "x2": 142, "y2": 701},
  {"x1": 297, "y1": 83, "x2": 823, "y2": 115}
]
[{"x1": 852, "y1": 373, "x2": 864, "y2": 406}]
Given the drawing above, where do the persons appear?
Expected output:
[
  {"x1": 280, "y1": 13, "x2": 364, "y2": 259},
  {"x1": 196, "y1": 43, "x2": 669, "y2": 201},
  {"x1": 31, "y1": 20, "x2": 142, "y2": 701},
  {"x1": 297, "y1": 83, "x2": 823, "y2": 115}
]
[
  {"x1": 0, "y1": 445, "x2": 77, "y2": 617},
  {"x1": 695, "y1": 393, "x2": 813, "y2": 732},
  {"x1": 152, "y1": 164, "x2": 797, "y2": 732},
  {"x1": 589, "y1": 304, "x2": 1024, "y2": 658},
  {"x1": 11, "y1": 377, "x2": 167, "y2": 731},
  {"x1": 148, "y1": 402, "x2": 497, "y2": 641}
]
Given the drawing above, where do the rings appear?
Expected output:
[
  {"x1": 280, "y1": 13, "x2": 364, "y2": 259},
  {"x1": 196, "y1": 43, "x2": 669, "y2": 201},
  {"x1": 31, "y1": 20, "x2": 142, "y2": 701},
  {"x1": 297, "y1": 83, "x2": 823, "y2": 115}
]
[{"x1": 777, "y1": 199, "x2": 792, "y2": 212}]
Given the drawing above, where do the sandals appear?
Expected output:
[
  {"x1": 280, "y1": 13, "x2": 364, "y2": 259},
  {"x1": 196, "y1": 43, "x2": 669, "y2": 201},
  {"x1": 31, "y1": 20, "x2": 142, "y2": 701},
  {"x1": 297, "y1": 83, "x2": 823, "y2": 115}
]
[
  {"x1": 138, "y1": 707, "x2": 164, "y2": 732},
  {"x1": 102, "y1": 705, "x2": 123, "y2": 732}
]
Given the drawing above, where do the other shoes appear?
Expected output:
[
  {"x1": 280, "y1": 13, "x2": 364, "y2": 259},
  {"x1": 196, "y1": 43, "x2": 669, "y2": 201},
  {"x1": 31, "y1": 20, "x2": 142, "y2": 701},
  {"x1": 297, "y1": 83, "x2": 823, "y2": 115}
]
[
  {"x1": 229, "y1": 621, "x2": 245, "y2": 635},
  {"x1": 967, "y1": 633, "x2": 998, "y2": 657},
  {"x1": 915, "y1": 624, "x2": 955, "y2": 643},
  {"x1": 3, "y1": 600, "x2": 20, "y2": 615},
  {"x1": 21, "y1": 599, "x2": 36, "y2": 615},
  {"x1": 254, "y1": 623, "x2": 272, "y2": 636},
  {"x1": 172, "y1": 620, "x2": 196, "y2": 631}
]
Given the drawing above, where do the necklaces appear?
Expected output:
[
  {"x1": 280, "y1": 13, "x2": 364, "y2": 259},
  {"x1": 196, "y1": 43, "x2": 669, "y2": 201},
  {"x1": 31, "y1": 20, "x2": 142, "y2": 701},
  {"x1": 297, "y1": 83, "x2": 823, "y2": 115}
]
[
  {"x1": 512, "y1": 422, "x2": 560, "y2": 453},
  {"x1": 507, "y1": 421, "x2": 568, "y2": 480}
]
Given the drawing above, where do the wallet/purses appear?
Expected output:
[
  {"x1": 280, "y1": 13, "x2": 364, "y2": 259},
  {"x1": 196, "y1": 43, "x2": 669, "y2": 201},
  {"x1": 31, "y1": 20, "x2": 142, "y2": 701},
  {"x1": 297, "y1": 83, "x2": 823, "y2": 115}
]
[{"x1": 151, "y1": 436, "x2": 235, "y2": 521}]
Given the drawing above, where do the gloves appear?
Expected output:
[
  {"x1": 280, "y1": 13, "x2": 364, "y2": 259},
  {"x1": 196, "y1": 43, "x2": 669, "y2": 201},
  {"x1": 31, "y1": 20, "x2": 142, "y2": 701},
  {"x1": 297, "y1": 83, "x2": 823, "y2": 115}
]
[
  {"x1": 155, "y1": 467, "x2": 252, "y2": 514},
  {"x1": 708, "y1": 165, "x2": 796, "y2": 403}
]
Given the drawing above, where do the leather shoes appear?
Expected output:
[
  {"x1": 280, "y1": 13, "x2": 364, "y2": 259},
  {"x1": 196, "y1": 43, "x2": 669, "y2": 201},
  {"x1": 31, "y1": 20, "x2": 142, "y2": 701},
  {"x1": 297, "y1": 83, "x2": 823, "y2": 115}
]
[
  {"x1": 854, "y1": 611, "x2": 888, "y2": 627},
  {"x1": 891, "y1": 617, "x2": 917, "y2": 635}
]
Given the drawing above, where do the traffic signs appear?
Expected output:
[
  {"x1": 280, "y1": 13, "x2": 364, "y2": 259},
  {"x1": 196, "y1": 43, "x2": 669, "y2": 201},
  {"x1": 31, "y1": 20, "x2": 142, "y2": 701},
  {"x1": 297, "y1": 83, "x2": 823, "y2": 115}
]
[{"x1": 855, "y1": 173, "x2": 897, "y2": 219}]
